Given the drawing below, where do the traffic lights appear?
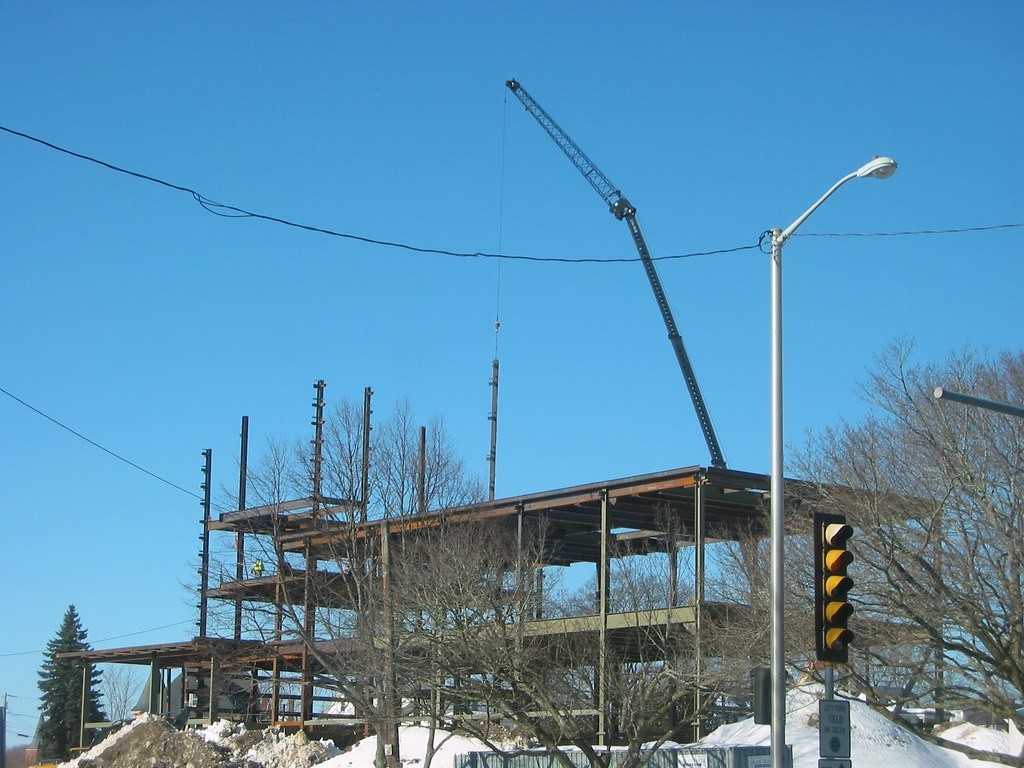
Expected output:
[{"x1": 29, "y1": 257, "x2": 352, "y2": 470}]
[{"x1": 813, "y1": 512, "x2": 854, "y2": 663}]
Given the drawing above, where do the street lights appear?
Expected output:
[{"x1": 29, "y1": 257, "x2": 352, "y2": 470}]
[{"x1": 769, "y1": 155, "x2": 897, "y2": 768}]
